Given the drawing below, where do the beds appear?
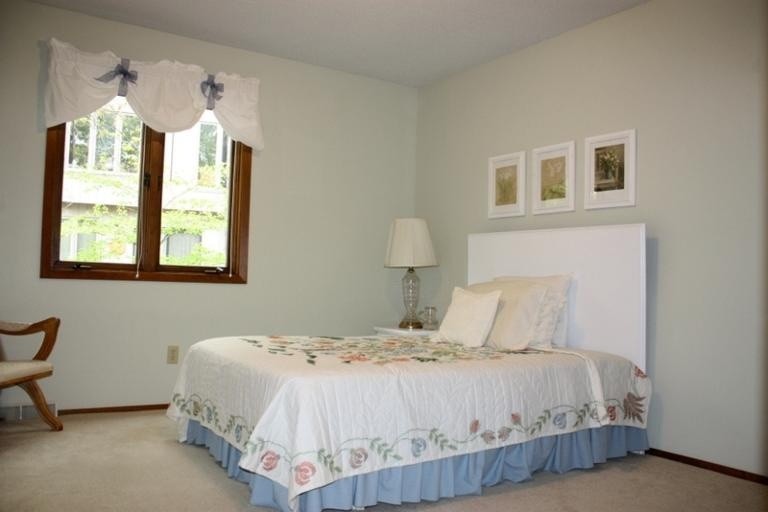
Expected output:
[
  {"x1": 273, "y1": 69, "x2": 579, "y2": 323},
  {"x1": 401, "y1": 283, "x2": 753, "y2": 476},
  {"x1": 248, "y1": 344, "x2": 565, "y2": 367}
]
[{"x1": 167, "y1": 223, "x2": 646, "y2": 512}]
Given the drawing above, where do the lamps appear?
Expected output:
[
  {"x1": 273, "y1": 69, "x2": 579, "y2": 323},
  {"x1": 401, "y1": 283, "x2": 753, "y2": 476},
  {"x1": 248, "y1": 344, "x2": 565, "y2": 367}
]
[{"x1": 384, "y1": 218, "x2": 438, "y2": 329}]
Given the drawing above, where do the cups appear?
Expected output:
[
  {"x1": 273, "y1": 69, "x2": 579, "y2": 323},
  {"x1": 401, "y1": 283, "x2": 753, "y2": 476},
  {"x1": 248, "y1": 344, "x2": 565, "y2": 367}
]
[{"x1": 419, "y1": 306, "x2": 437, "y2": 325}]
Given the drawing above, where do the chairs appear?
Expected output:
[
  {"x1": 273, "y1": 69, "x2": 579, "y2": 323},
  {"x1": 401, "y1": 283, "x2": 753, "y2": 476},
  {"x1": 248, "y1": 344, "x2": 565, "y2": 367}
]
[{"x1": 0, "y1": 317, "x2": 63, "y2": 431}]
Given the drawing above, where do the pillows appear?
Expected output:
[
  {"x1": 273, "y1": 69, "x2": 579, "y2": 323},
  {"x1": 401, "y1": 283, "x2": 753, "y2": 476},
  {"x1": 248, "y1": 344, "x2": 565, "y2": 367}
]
[{"x1": 431, "y1": 277, "x2": 574, "y2": 353}]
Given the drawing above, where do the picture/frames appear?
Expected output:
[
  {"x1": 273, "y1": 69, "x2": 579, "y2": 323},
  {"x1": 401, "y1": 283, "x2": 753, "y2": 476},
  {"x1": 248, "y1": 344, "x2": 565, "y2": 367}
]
[
  {"x1": 531, "y1": 141, "x2": 575, "y2": 215},
  {"x1": 488, "y1": 151, "x2": 526, "y2": 220},
  {"x1": 584, "y1": 130, "x2": 637, "y2": 210}
]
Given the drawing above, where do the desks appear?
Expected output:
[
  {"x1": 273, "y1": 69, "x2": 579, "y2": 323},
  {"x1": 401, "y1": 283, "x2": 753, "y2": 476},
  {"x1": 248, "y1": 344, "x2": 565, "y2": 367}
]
[{"x1": 373, "y1": 325, "x2": 438, "y2": 335}]
